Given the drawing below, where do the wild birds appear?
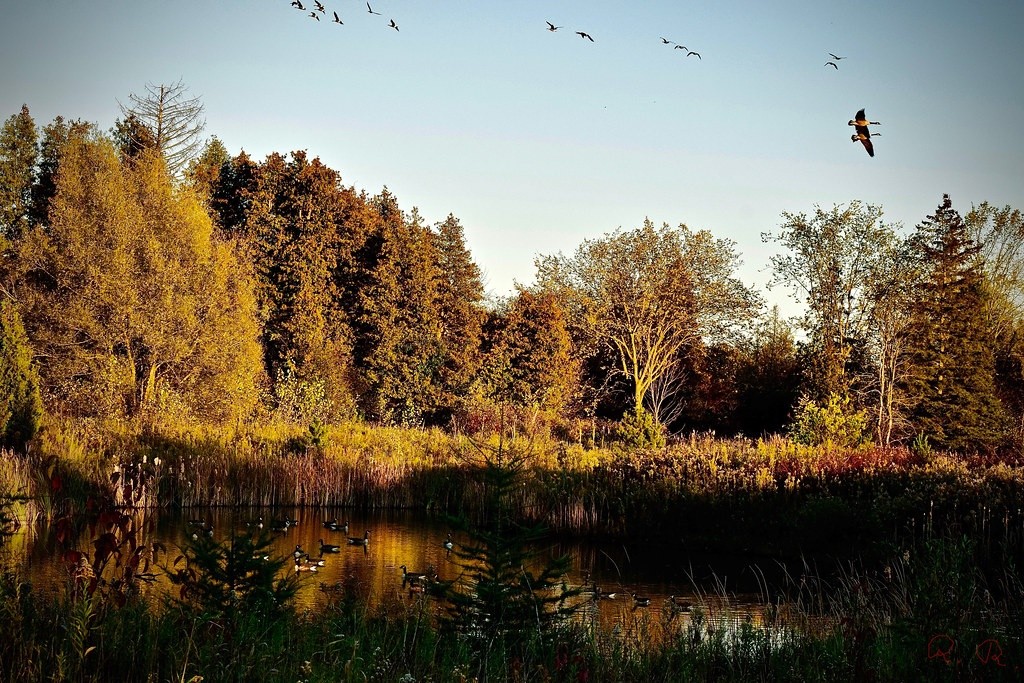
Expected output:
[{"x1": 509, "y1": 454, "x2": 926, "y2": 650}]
[
  {"x1": 387, "y1": 19, "x2": 400, "y2": 32},
  {"x1": 546, "y1": 20, "x2": 564, "y2": 33},
  {"x1": 847, "y1": 107, "x2": 883, "y2": 158},
  {"x1": 824, "y1": 61, "x2": 839, "y2": 71},
  {"x1": 289, "y1": 0, "x2": 344, "y2": 26},
  {"x1": 574, "y1": 31, "x2": 595, "y2": 43},
  {"x1": 366, "y1": 1, "x2": 383, "y2": 16},
  {"x1": 190, "y1": 517, "x2": 453, "y2": 593},
  {"x1": 828, "y1": 52, "x2": 847, "y2": 61},
  {"x1": 580, "y1": 572, "x2": 697, "y2": 618},
  {"x1": 659, "y1": 36, "x2": 701, "y2": 60}
]
